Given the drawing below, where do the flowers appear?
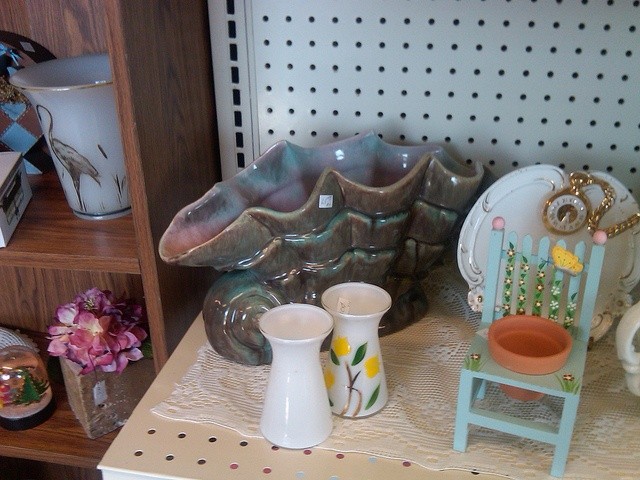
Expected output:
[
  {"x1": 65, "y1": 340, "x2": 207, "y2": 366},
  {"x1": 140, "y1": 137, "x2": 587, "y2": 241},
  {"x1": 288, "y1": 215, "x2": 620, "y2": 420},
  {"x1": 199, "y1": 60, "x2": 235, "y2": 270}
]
[{"x1": 44, "y1": 285, "x2": 152, "y2": 373}]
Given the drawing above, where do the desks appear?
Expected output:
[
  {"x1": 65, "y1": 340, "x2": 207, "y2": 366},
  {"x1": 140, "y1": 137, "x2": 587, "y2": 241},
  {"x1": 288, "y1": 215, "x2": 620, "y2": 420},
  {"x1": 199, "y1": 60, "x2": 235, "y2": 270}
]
[{"x1": 95, "y1": 261, "x2": 640, "y2": 480}]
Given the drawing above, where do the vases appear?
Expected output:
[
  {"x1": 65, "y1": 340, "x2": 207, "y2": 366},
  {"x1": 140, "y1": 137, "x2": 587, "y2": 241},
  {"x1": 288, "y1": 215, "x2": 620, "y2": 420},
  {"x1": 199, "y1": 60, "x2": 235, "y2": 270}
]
[{"x1": 59, "y1": 356, "x2": 153, "y2": 440}]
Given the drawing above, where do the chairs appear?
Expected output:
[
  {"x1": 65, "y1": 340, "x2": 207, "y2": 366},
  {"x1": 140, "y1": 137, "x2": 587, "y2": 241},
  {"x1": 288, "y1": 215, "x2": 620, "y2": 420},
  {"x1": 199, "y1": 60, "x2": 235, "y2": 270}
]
[{"x1": 453, "y1": 218, "x2": 528, "y2": 298}]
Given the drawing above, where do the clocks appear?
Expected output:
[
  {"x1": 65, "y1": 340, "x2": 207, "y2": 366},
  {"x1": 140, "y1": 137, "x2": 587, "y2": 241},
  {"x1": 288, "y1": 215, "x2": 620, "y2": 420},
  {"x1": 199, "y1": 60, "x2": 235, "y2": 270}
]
[{"x1": 541, "y1": 189, "x2": 591, "y2": 235}]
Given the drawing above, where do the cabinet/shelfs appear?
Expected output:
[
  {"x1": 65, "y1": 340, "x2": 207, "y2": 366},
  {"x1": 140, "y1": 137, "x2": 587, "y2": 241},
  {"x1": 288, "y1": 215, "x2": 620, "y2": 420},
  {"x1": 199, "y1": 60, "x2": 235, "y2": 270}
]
[{"x1": 0, "y1": 0, "x2": 219, "y2": 477}]
